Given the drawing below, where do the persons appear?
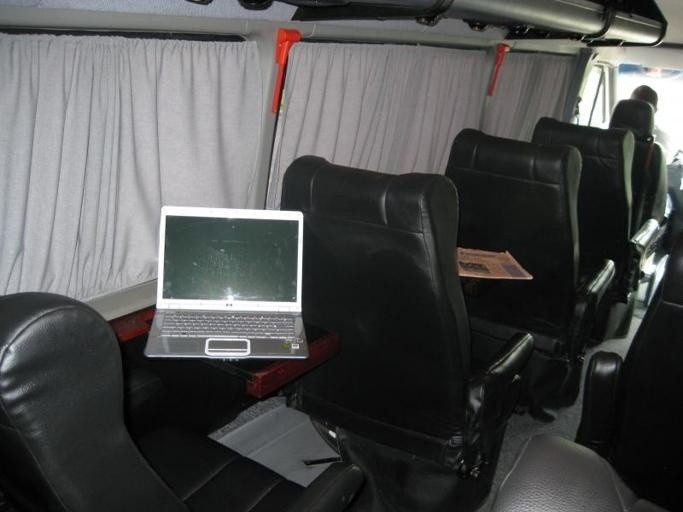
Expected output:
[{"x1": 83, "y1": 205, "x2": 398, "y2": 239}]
[{"x1": 628, "y1": 84, "x2": 669, "y2": 153}]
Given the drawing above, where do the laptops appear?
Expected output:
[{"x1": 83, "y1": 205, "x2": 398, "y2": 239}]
[{"x1": 143, "y1": 205, "x2": 310, "y2": 360}]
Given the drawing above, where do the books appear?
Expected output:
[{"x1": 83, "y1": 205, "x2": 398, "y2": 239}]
[{"x1": 455, "y1": 247, "x2": 534, "y2": 281}]
